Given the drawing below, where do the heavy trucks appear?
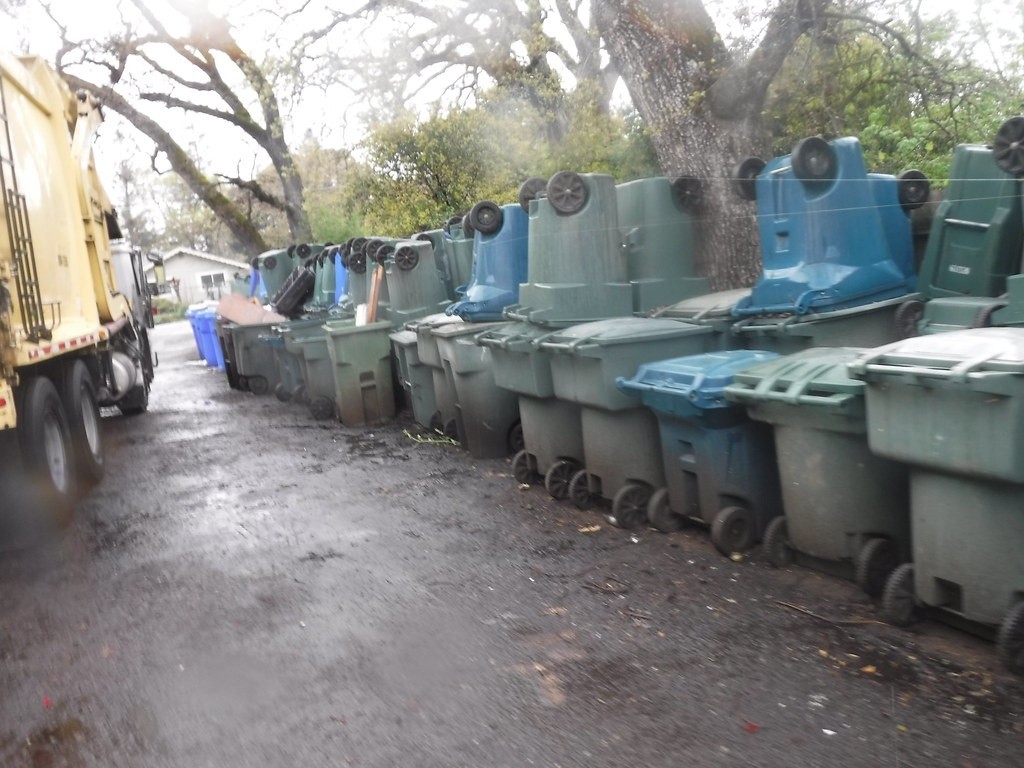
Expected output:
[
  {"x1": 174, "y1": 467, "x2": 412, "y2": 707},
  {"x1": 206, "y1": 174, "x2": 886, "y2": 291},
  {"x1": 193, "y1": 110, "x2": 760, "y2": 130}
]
[{"x1": 0, "y1": 50, "x2": 164, "y2": 534}]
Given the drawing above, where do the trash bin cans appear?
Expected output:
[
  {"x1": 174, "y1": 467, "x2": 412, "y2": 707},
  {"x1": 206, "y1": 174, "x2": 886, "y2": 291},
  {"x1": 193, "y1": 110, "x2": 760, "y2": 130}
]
[
  {"x1": 615, "y1": 348, "x2": 788, "y2": 555},
  {"x1": 533, "y1": 312, "x2": 715, "y2": 527},
  {"x1": 850, "y1": 325, "x2": 1023, "y2": 669},
  {"x1": 725, "y1": 347, "x2": 911, "y2": 589},
  {"x1": 230, "y1": 168, "x2": 750, "y2": 455},
  {"x1": 183, "y1": 300, "x2": 227, "y2": 372},
  {"x1": 735, "y1": 138, "x2": 930, "y2": 356},
  {"x1": 910, "y1": 112, "x2": 1023, "y2": 330}
]
